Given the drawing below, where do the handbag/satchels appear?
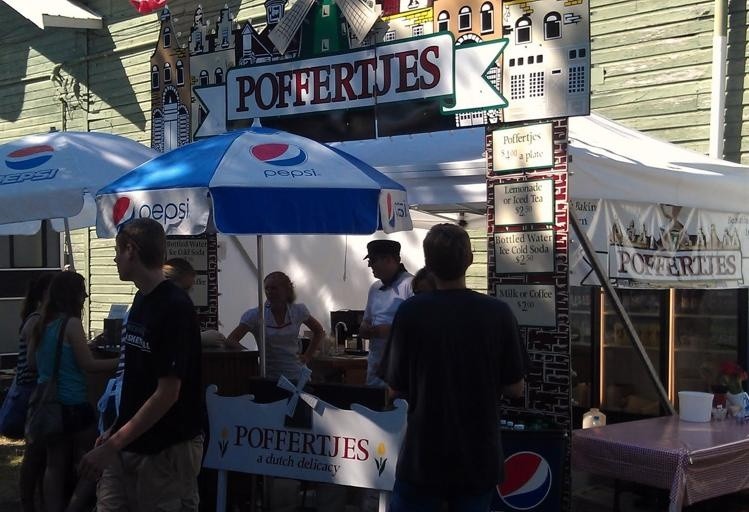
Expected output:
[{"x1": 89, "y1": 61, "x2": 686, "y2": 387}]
[
  {"x1": 24, "y1": 314, "x2": 74, "y2": 444},
  {"x1": 0, "y1": 369, "x2": 38, "y2": 440}
]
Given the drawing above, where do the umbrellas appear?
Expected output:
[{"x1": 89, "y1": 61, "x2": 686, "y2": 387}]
[
  {"x1": 96, "y1": 116, "x2": 415, "y2": 378},
  {"x1": 1, "y1": 125, "x2": 161, "y2": 281}
]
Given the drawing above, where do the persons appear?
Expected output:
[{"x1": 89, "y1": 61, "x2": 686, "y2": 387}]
[
  {"x1": 411, "y1": 267, "x2": 436, "y2": 295},
  {"x1": 36, "y1": 271, "x2": 120, "y2": 512},
  {"x1": 226, "y1": 271, "x2": 325, "y2": 381},
  {"x1": 358, "y1": 240, "x2": 415, "y2": 387},
  {"x1": 77, "y1": 218, "x2": 205, "y2": 512},
  {"x1": 376, "y1": 223, "x2": 526, "y2": 512},
  {"x1": 97, "y1": 257, "x2": 226, "y2": 445},
  {"x1": 18, "y1": 271, "x2": 51, "y2": 512}
]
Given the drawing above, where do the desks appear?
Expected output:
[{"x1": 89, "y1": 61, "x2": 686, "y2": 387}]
[{"x1": 571, "y1": 414, "x2": 749, "y2": 512}]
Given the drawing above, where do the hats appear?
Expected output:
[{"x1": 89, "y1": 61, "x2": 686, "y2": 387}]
[{"x1": 363, "y1": 240, "x2": 401, "y2": 260}]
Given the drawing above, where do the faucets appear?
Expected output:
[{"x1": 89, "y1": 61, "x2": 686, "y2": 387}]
[{"x1": 335, "y1": 320, "x2": 347, "y2": 357}]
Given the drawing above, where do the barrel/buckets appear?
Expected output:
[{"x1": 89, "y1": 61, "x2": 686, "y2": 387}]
[{"x1": 677, "y1": 391, "x2": 714, "y2": 424}]
